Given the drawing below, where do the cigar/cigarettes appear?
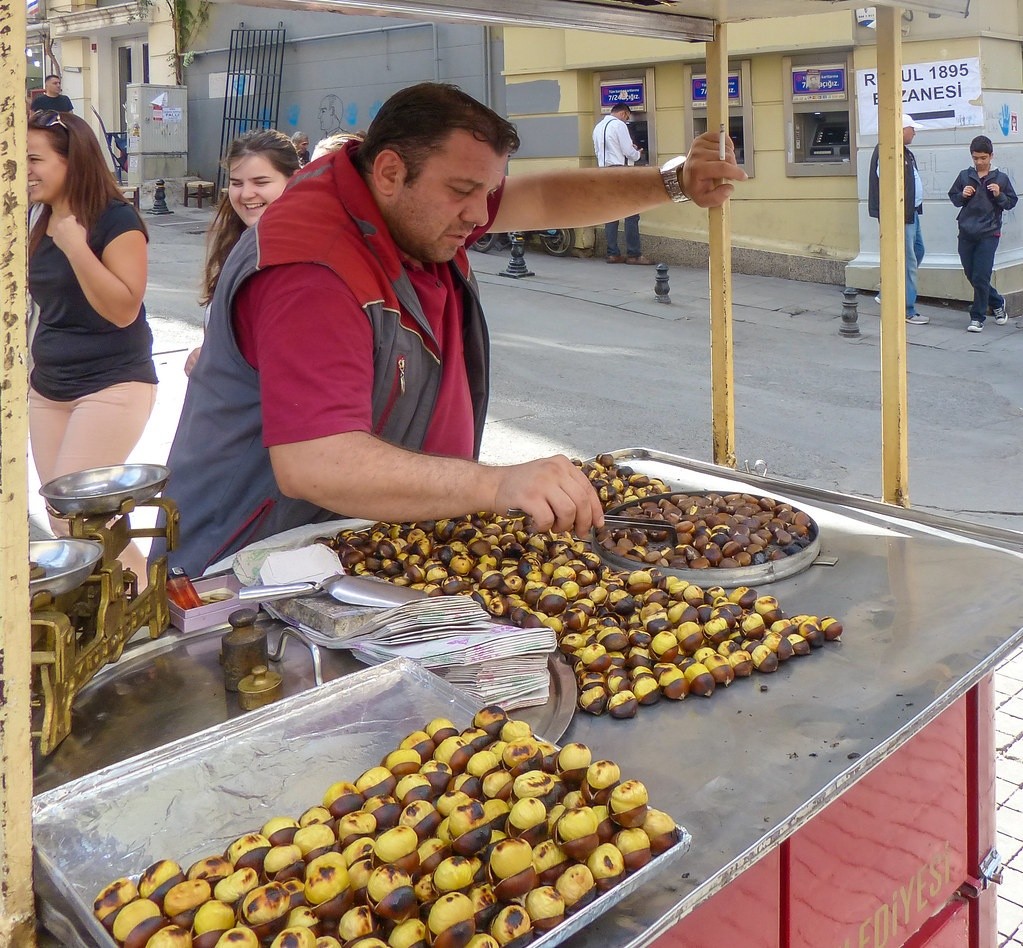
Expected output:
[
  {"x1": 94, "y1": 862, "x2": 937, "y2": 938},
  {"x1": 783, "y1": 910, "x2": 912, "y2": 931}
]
[{"x1": 720, "y1": 123, "x2": 726, "y2": 161}]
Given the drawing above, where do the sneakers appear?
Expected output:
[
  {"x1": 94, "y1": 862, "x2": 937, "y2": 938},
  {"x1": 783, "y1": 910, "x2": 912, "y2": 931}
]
[
  {"x1": 993, "y1": 299, "x2": 1008, "y2": 325},
  {"x1": 967, "y1": 320, "x2": 984, "y2": 333},
  {"x1": 905, "y1": 313, "x2": 930, "y2": 325},
  {"x1": 875, "y1": 294, "x2": 881, "y2": 304}
]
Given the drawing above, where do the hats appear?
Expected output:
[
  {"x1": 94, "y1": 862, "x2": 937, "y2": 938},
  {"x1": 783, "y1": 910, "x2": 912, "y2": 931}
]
[{"x1": 902, "y1": 114, "x2": 924, "y2": 129}]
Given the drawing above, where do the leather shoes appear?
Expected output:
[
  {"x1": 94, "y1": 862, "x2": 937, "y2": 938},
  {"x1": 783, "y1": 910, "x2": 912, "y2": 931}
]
[
  {"x1": 626, "y1": 255, "x2": 656, "y2": 264},
  {"x1": 607, "y1": 255, "x2": 626, "y2": 263}
]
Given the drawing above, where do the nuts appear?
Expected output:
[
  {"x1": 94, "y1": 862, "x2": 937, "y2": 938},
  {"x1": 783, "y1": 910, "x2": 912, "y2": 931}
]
[
  {"x1": 315, "y1": 512, "x2": 843, "y2": 720},
  {"x1": 596, "y1": 493, "x2": 811, "y2": 568},
  {"x1": 93, "y1": 706, "x2": 681, "y2": 948},
  {"x1": 571, "y1": 453, "x2": 671, "y2": 509}
]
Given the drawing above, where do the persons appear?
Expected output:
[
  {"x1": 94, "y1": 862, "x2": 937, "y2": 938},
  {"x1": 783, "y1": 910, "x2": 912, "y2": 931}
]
[
  {"x1": 30, "y1": 75, "x2": 73, "y2": 114},
  {"x1": 869, "y1": 114, "x2": 932, "y2": 324},
  {"x1": 592, "y1": 103, "x2": 656, "y2": 266},
  {"x1": 154, "y1": 82, "x2": 748, "y2": 573},
  {"x1": 288, "y1": 132, "x2": 309, "y2": 167},
  {"x1": 180, "y1": 128, "x2": 301, "y2": 376},
  {"x1": 26, "y1": 108, "x2": 157, "y2": 539},
  {"x1": 947, "y1": 135, "x2": 1018, "y2": 332}
]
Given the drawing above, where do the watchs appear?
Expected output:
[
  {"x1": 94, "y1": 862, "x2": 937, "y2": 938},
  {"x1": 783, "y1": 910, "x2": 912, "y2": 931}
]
[{"x1": 659, "y1": 156, "x2": 690, "y2": 203}]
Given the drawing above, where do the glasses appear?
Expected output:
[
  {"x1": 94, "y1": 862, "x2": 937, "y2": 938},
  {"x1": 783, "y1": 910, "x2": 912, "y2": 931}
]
[
  {"x1": 298, "y1": 141, "x2": 309, "y2": 145},
  {"x1": 33, "y1": 109, "x2": 68, "y2": 130},
  {"x1": 625, "y1": 112, "x2": 629, "y2": 120}
]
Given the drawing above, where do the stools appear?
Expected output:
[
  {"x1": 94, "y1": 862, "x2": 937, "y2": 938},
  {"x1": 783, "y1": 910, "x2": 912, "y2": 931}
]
[
  {"x1": 117, "y1": 186, "x2": 139, "y2": 212},
  {"x1": 185, "y1": 180, "x2": 216, "y2": 209}
]
